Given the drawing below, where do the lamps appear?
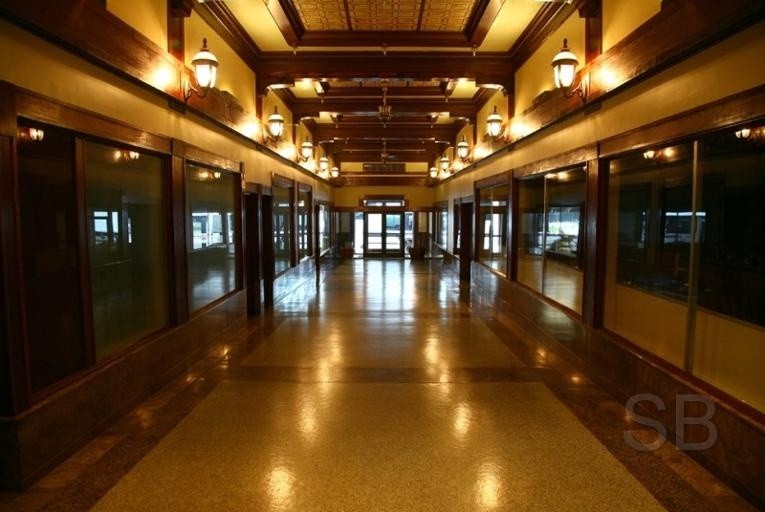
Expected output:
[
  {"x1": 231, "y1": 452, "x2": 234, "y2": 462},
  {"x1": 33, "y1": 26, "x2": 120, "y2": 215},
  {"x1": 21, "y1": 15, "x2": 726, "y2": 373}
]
[
  {"x1": 17, "y1": 125, "x2": 44, "y2": 150},
  {"x1": 261, "y1": 105, "x2": 343, "y2": 183},
  {"x1": 429, "y1": 106, "x2": 510, "y2": 180},
  {"x1": 183, "y1": 37, "x2": 219, "y2": 104},
  {"x1": 550, "y1": 37, "x2": 588, "y2": 102},
  {"x1": 643, "y1": 142, "x2": 683, "y2": 164},
  {"x1": 735, "y1": 126, "x2": 765, "y2": 140},
  {"x1": 197, "y1": 167, "x2": 224, "y2": 183},
  {"x1": 112, "y1": 148, "x2": 139, "y2": 168}
]
